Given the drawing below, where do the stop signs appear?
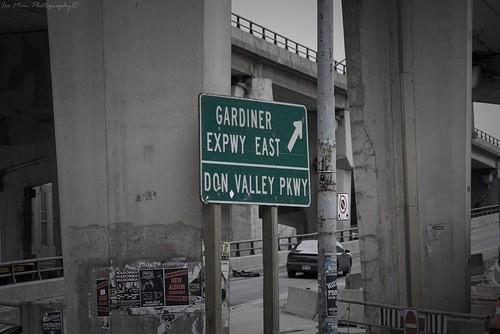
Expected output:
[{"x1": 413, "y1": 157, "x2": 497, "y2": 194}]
[{"x1": 403, "y1": 308, "x2": 419, "y2": 334}]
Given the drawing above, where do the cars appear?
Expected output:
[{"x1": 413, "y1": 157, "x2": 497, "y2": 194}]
[{"x1": 286, "y1": 239, "x2": 352, "y2": 278}]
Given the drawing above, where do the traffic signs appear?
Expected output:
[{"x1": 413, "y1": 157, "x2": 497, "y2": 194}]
[{"x1": 199, "y1": 94, "x2": 311, "y2": 208}]
